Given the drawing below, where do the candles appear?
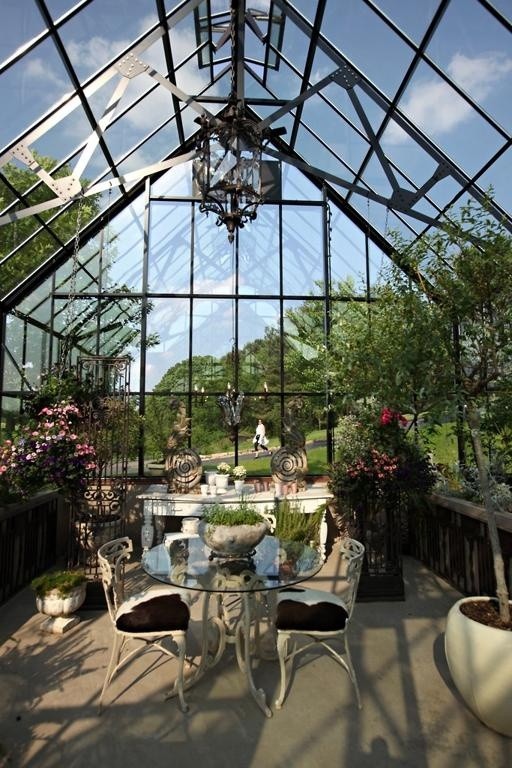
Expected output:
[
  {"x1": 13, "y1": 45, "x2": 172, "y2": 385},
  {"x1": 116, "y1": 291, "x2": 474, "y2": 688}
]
[{"x1": 193, "y1": 382, "x2": 270, "y2": 392}]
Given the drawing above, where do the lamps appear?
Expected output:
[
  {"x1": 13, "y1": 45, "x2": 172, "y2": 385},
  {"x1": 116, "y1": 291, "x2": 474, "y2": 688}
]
[{"x1": 193, "y1": 0, "x2": 261, "y2": 241}]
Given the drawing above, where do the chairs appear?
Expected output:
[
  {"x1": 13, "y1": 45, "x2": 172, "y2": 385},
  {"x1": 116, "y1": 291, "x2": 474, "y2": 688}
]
[
  {"x1": 197, "y1": 516, "x2": 277, "y2": 536},
  {"x1": 97, "y1": 536, "x2": 191, "y2": 714},
  {"x1": 274, "y1": 536, "x2": 365, "y2": 711}
]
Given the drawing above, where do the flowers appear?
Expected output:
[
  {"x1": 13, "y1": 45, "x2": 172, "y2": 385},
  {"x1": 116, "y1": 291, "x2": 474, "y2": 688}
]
[
  {"x1": 1, "y1": 400, "x2": 97, "y2": 496},
  {"x1": 216, "y1": 461, "x2": 247, "y2": 480}
]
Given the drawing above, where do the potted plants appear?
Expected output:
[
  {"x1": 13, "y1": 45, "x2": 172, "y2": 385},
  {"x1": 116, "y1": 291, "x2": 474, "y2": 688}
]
[
  {"x1": 198, "y1": 491, "x2": 332, "y2": 580},
  {"x1": 31, "y1": 571, "x2": 87, "y2": 632},
  {"x1": 301, "y1": 187, "x2": 512, "y2": 739}
]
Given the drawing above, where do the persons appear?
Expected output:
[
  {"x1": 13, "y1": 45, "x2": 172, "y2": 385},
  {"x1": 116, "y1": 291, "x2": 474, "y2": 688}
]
[{"x1": 252, "y1": 418, "x2": 273, "y2": 458}]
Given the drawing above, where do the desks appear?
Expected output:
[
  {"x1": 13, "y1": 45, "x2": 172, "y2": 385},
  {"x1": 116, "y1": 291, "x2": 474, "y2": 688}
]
[{"x1": 141, "y1": 536, "x2": 324, "y2": 718}]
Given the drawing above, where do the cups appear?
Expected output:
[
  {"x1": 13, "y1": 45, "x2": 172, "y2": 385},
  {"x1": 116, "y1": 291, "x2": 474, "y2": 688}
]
[
  {"x1": 210, "y1": 485, "x2": 217, "y2": 496},
  {"x1": 200, "y1": 484, "x2": 209, "y2": 497}
]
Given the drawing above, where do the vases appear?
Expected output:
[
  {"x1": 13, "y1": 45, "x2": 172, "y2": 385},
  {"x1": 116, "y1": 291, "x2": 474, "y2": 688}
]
[
  {"x1": 69, "y1": 516, "x2": 123, "y2": 567},
  {"x1": 203, "y1": 471, "x2": 245, "y2": 494}
]
